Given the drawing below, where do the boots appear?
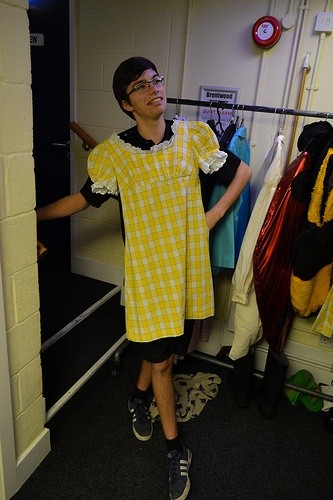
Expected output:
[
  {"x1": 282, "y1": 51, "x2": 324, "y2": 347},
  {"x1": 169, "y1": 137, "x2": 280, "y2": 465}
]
[
  {"x1": 251, "y1": 346, "x2": 289, "y2": 419},
  {"x1": 228, "y1": 346, "x2": 256, "y2": 409}
]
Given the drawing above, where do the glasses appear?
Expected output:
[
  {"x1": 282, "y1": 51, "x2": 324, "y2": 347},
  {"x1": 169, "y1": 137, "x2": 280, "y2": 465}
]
[{"x1": 127, "y1": 76, "x2": 165, "y2": 96}]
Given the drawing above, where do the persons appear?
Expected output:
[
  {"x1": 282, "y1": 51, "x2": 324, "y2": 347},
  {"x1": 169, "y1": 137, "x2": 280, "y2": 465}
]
[{"x1": 36, "y1": 57, "x2": 253, "y2": 500}]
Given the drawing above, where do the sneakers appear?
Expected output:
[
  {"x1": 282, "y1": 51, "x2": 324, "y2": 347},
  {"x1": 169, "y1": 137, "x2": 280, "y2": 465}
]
[
  {"x1": 128, "y1": 399, "x2": 153, "y2": 441},
  {"x1": 167, "y1": 446, "x2": 192, "y2": 500}
]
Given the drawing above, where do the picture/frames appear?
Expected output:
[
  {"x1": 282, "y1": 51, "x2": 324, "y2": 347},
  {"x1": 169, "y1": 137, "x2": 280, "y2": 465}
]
[{"x1": 198, "y1": 85, "x2": 241, "y2": 136}]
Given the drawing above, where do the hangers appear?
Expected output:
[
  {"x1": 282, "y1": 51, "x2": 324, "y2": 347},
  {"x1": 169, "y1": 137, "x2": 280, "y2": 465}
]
[
  {"x1": 277, "y1": 108, "x2": 285, "y2": 136},
  {"x1": 264, "y1": 107, "x2": 278, "y2": 158},
  {"x1": 210, "y1": 100, "x2": 244, "y2": 137}
]
